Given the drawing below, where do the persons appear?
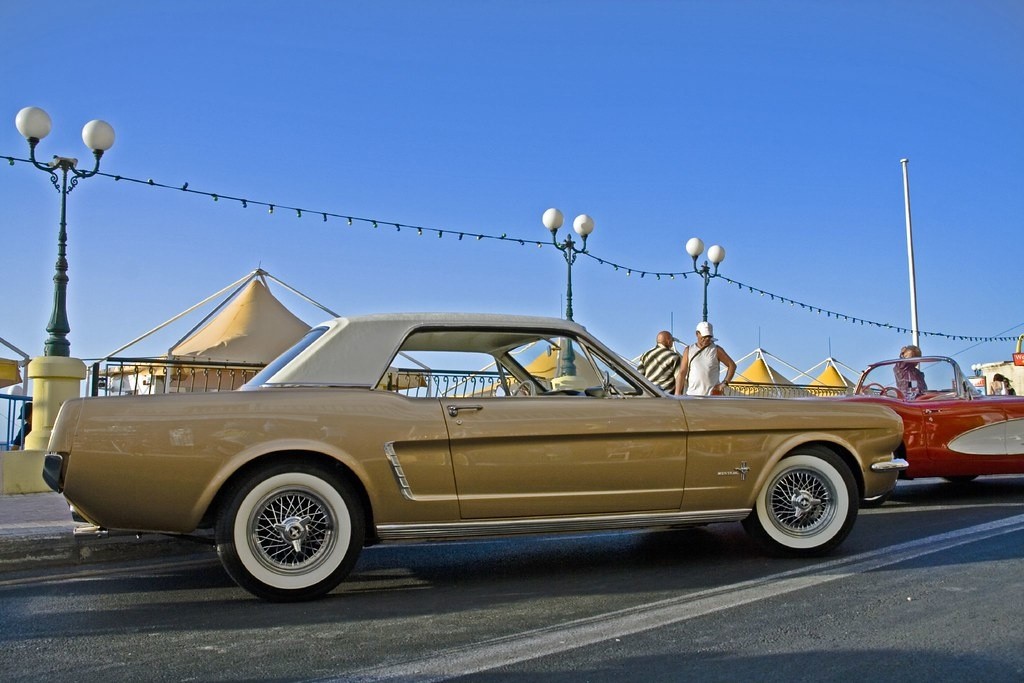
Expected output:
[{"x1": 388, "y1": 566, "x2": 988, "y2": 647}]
[
  {"x1": 637, "y1": 330, "x2": 684, "y2": 395},
  {"x1": 10, "y1": 402, "x2": 32, "y2": 450},
  {"x1": 893, "y1": 345, "x2": 928, "y2": 390},
  {"x1": 674, "y1": 322, "x2": 737, "y2": 396},
  {"x1": 990, "y1": 373, "x2": 1016, "y2": 395}
]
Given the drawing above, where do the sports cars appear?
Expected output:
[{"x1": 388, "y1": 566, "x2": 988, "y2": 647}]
[{"x1": 712, "y1": 356, "x2": 1024, "y2": 509}]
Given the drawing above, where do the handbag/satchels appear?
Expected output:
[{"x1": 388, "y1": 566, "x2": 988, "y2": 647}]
[{"x1": 1001, "y1": 381, "x2": 1009, "y2": 396}]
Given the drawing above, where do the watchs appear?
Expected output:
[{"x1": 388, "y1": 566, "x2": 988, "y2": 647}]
[{"x1": 722, "y1": 379, "x2": 728, "y2": 386}]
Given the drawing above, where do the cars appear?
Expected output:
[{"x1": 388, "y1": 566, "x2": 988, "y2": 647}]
[{"x1": 40, "y1": 312, "x2": 905, "y2": 603}]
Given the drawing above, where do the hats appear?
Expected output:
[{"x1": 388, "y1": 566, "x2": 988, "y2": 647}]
[{"x1": 696, "y1": 322, "x2": 713, "y2": 337}]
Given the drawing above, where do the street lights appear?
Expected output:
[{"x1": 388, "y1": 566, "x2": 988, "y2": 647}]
[
  {"x1": 686, "y1": 237, "x2": 725, "y2": 322},
  {"x1": 16, "y1": 106, "x2": 115, "y2": 357},
  {"x1": 542, "y1": 207, "x2": 595, "y2": 377}
]
